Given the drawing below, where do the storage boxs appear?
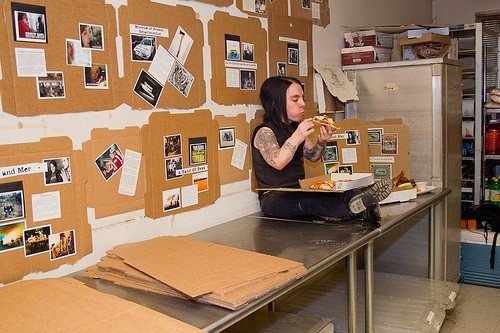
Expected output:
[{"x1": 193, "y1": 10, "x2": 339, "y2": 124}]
[
  {"x1": 378, "y1": 188, "x2": 417, "y2": 205},
  {"x1": 255, "y1": 172, "x2": 375, "y2": 195},
  {"x1": 461, "y1": 229, "x2": 500, "y2": 288},
  {"x1": 340, "y1": 27, "x2": 458, "y2": 66}
]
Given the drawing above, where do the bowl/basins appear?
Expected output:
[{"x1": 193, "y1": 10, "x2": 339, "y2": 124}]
[{"x1": 416, "y1": 182, "x2": 427, "y2": 190}]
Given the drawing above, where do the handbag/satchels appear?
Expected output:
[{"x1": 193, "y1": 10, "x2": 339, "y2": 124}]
[{"x1": 474, "y1": 203, "x2": 500, "y2": 233}]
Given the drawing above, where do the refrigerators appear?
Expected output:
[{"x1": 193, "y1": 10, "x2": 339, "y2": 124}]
[{"x1": 341, "y1": 58, "x2": 462, "y2": 283}]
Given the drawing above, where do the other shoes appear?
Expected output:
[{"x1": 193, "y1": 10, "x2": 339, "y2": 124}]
[
  {"x1": 346, "y1": 177, "x2": 394, "y2": 214},
  {"x1": 360, "y1": 203, "x2": 382, "y2": 221}
]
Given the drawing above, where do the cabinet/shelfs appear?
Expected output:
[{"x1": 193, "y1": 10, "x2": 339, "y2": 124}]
[{"x1": 452, "y1": 23, "x2": 500, "y2": 207}]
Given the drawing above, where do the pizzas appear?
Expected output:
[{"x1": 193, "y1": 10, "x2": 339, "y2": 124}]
[
  {"x1": 312, "y1": 114, "x2": 338, "y2": 130},
  {"x1": 309, "y1": 179, "x2": 354, "y2": 190}
]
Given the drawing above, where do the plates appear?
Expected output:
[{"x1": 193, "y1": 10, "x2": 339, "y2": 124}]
[{"x1": 417, "y1": 186, "x2": 436, "y2": 194}]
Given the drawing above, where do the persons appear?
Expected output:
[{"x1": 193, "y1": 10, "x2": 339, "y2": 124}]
[
  {"x1": 129, "y1": 0, "x2": 399, "y2": 214},
  {"x1": 8, "y1": 0, "x2": 108, "y2": 99},
  {"x1": 0, "y1": 136, "x2": 127, "y2": 260},
  {"x1": 249, "y1": 75, "x2": 394, "y2": 223}
]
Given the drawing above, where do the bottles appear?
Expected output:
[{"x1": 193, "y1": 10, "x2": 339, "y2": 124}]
[
  {"x1": 485, "y1": 120, "x2": 500, "y2": 155},
  {"x1": 485, "y1": 178, "x2": 491, "y2": 203},
  {"x1": 492, "y1": 176, "x2": 500, "y2": 207},
  {"x1": 461, "y1": 176, "x2": 474, "y2": 200}
]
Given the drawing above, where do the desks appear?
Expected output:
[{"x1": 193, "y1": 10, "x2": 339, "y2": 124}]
[
  {"x1": 66, "y1": 216, "x2": 378, "y2": 333},
  {"x1": 249, "y1": 188, "x2": 452, "y2": 333}
]
